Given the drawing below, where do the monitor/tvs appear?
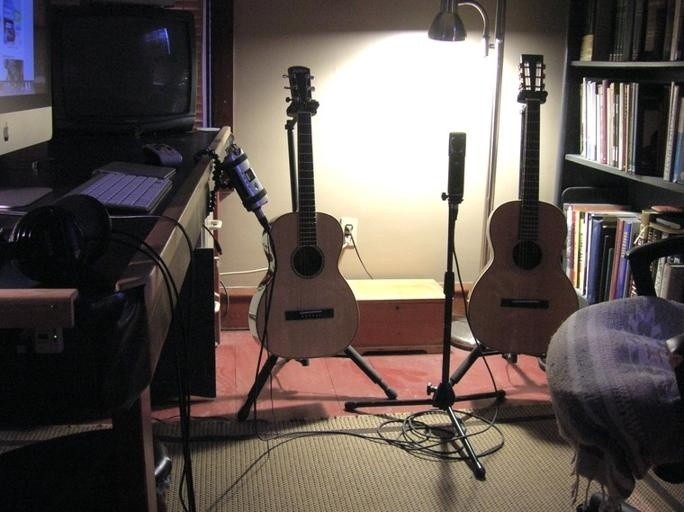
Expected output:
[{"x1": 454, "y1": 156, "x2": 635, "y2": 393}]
[
  {"x1": 0, "y1": 0, "x2": 54, "y2": 208},
  {"x1": 50, "y1": 4, "x2": 199, "y2": 136}
]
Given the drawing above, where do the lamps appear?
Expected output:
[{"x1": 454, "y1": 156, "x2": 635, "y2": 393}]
[{"x1": 425, "y1": 0, "x2": 509, "y2": 352}]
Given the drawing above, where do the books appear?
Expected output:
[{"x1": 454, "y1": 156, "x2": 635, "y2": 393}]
[{"x1": 559, "y1": 0, "x2": 684, "y2": 305}]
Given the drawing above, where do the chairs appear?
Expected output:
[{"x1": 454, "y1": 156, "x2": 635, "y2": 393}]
[{"x1": 545, "y1": 236, "x2": 682, "y2": 508}]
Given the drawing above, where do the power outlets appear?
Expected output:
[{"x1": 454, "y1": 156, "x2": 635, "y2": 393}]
[{"x1": 339, "y1": 216, "x2": 359, "y2": 248}]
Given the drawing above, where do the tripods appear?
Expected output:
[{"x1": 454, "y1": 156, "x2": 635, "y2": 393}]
[
  {"x1": 344, "y1": 201, "x2": 505, "y2": 482},
  {"x1": 235, "y1": 117, "x2": 397, "y2": 420}
]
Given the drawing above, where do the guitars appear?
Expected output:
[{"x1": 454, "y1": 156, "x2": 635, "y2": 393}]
[
  {"x1": 466, "y1": 54, "x2": 579, "y2": 355},
  {"x1": 247, "y1": 66, "x2": 360, "y2": 357}
]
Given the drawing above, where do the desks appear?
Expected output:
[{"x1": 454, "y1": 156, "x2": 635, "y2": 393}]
[{"x1": 0, "y1": 124, "x2": 229, "y2": 510}]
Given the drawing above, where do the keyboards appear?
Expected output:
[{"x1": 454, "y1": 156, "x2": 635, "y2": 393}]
[{"x1": 55, "y1": 171, "x2": 175, "y2": 214}]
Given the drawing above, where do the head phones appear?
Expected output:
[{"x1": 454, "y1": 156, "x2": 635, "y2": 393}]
[{"x1": 0, "y1": 193, "x2": 113, "y2": 284}]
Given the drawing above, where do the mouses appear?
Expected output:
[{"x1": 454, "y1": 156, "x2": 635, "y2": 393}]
[{"x1": 141, "y1": 141, "x2": 184, "y2": 167}]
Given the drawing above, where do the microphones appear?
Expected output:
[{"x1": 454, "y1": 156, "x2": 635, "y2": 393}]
[{"x1": 447, "y1": 132, "x2": 466, "y2": 203}]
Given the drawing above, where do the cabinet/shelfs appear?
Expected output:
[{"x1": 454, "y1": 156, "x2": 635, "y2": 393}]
[{"x1": 551, "y1": 0, "x2": 684, "y2": 309}]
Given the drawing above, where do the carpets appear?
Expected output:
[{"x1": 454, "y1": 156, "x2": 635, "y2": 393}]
[{"x1": 89, "y1": 329, "x2": 556, "y2": 422}]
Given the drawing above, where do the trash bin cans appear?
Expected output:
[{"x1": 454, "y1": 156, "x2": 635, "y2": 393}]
[{"x1": 0, "y1": 428, "x2": 172, "y2": 512}]
